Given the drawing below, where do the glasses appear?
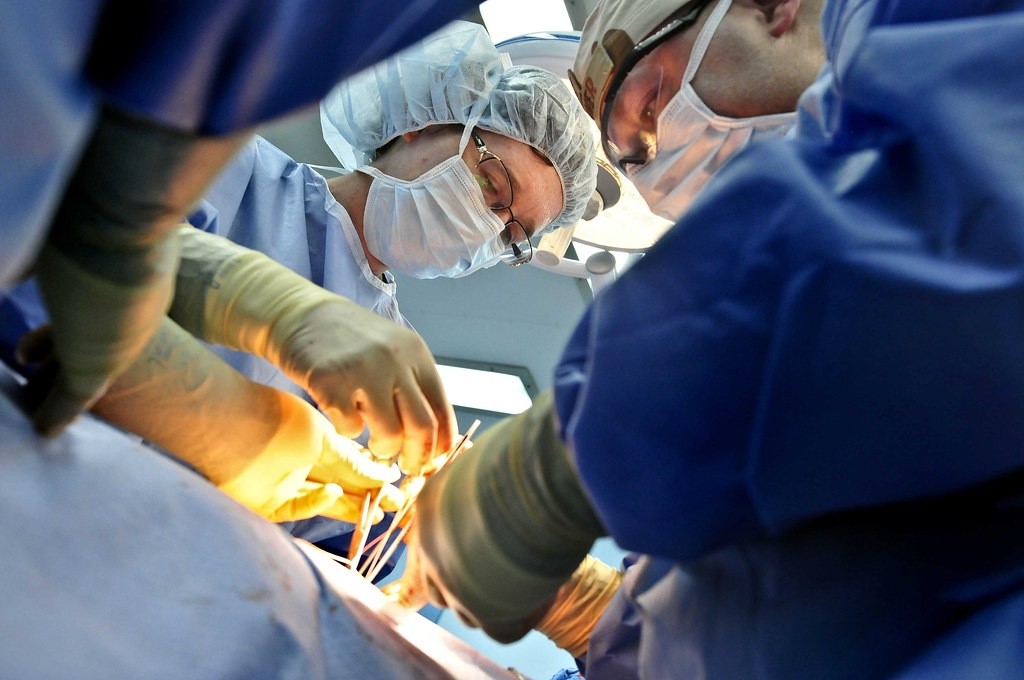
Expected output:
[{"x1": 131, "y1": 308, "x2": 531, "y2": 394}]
[
  {"x1": 601, "y1": 3, "x2": 710, "y2": 181},
  {"x1": 468, "y1": 125, "x2": 535, "y2": 269}
]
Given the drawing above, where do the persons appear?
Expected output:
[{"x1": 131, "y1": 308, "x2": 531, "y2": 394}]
[{"x1": 0, "y1": 1, "x2": 1024, "y2": 679}]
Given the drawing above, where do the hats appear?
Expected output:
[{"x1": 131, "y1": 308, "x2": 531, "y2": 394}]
[{"x1": 564, "y1": 1, "x2": 697, "y2": 132}]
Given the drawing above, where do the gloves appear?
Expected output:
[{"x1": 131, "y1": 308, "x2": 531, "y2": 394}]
[
  {"x1": 170, "y1": 230, "x2": 455, "y2": 478},
  {"x1": 536, "y1": 547, "x2": 632, "y2": 661},
  {"x1": 18, "y1": 116, "x2": 247, "y2": 440},
  {"x1": 95, "y1": 316, "x2": 408, "y2": 525},
  {"x1": 414, "y1": 389, "x2": 609, "y2": 641}
]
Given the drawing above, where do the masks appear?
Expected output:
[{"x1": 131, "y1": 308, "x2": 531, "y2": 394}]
[
  {"x1": 625, "y1": 0, "x2": 807, "y2": 225},
  {"x1": 352, "y1": 71, "x2": 506, "y2": 282}
]
